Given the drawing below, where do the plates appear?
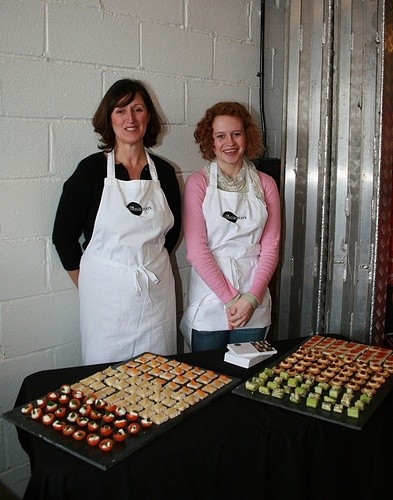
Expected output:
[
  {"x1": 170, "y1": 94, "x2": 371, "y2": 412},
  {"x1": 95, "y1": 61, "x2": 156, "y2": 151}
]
[
  {"x1": 232, "y1": 335, "x2": 393, "y2": 432},
  {"x1": 2, "y1": 351, "x2": 241, "y2": 472}
]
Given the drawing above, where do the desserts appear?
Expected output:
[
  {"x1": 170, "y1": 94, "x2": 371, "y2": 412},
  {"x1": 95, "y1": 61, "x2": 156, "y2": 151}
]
[
  {"x1": 245, "y1": 335, "x2": 393, "y2": 418},
  {"x1": 19, "y1": 353, "x2": 232, "y2": 452}
]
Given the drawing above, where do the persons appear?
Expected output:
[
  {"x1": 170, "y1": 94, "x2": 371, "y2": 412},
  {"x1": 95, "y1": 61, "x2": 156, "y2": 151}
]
[
  {"x1": 52, "y1": 77, "x2": 182, "y2": 365},
  {"x1": 178, "y1": 100, "x2": 281, "y2": 353}
]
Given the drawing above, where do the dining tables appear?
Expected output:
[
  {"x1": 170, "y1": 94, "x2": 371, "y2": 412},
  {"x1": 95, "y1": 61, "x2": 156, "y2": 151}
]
[{"x1": 10, "y1": 332, "x2": 393, "y2": 499}]
